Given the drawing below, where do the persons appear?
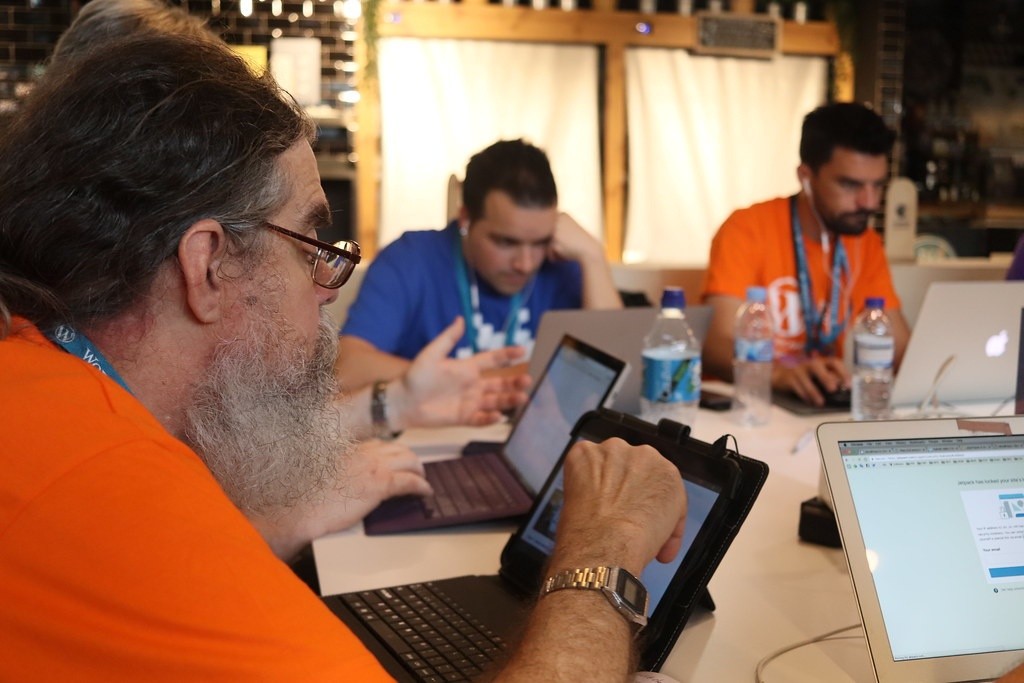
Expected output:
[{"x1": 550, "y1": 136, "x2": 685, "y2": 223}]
[
  {"x1": 0, "y1": 0, "x2": 686, "y2": 683},
  {"x1": 332, "y1": 139, "x2": 624, "y2": 393},
  {"x1": 700, "y1": 103, "x2": 911, "y2": 410}
]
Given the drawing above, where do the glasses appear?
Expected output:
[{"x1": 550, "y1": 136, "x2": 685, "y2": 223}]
[{"x1": 261, "y1": 221, "x2": 361, "y2": 290}]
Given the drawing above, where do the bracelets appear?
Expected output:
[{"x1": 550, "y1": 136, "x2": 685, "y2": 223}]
[{"x1": 372, "y1": 381, "x2": 403, "y2": 441}]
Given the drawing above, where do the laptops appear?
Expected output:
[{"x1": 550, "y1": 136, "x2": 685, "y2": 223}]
[
  {"x1": 321, "y1": 302, "x2": 769, "y2": 683},
  {"x1": 813, "y1": 277, "x2": 1024, "y2": 683}
]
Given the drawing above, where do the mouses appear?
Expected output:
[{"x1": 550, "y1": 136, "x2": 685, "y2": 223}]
[{"x1": 794, "y1": 375, "x2": 851, "y2": 411}]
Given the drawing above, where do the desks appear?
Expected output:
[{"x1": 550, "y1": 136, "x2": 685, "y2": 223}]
[
  {"x1": 310, "y1": 383, "x2": 1016, "y2": 683},
  {"x1": 920, "y1": 200, "x2": 1024, "y2": 227}
]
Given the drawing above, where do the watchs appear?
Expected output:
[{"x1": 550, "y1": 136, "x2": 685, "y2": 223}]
[{"x1": 540, "y1": 566, "x2": 649, "y2": 641}]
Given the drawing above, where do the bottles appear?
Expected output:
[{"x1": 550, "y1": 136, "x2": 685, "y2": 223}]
[
  {"x1": 638, "y1": 287, "x2": 702, "y2": 435},
  {"x1": 731, "y1": 288, "x2": 772, "y2": 426},
  {"x1": 851, "y1": 296, "x2": 895, "y2": 421}
]
